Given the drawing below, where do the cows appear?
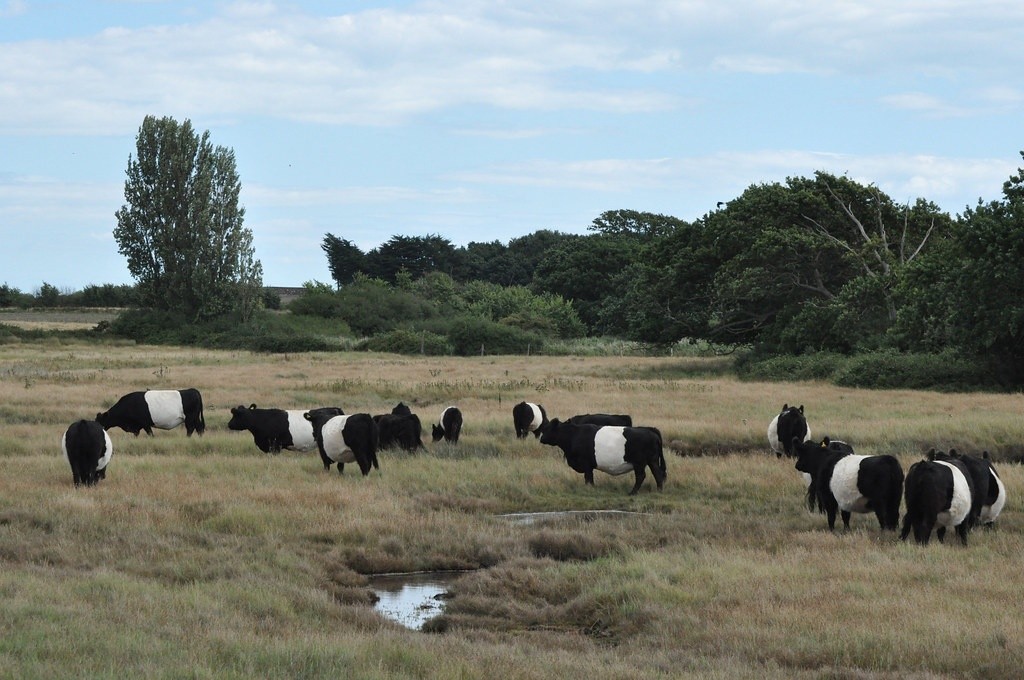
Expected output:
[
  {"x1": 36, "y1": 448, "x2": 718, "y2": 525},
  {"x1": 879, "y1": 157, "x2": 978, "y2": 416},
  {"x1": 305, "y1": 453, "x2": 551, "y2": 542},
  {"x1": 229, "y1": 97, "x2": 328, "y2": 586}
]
[
  {"x1": 227, "y1": 402, "x2": 464, "y2": 477},
  {"x1": 61, "y1": 388, "x2": 206, "y2": 490},
  {"x1": 513, "y1": 400, "x2": 667, "y2": 495},
  {"x1": 766, "y1": 403, "x2": 1006, "y2": 547}
]
